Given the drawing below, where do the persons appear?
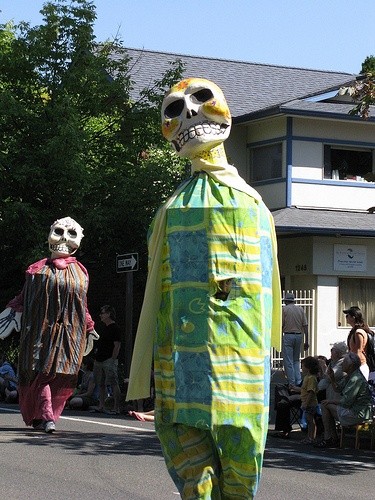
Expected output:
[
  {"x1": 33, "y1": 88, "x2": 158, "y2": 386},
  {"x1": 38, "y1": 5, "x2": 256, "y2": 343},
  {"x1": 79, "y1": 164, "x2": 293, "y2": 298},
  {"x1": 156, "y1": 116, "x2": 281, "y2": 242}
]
[
  {"x1": 0, "y1": 217, "x2": 96, "y2": 432},
  {"x1": 67, "y1": 356, "x2": 97, "y2": 411},
  {"x1": 128, "y1": 379, "x2": 156, "y2": 421},
  {"x1": 127, "y1": 79, "x2": 283, "y2": 500},
  {"x1": 343, "y1": 307, "x2": 369, "y2": 381},
  {"x1": 281, "y1": 293, "x2": 309, "y2": 387},
  {"x1": 0, "y1": 352, "x2": 19, "y2": 402},
  {"x1": 276, "y1": 342, "x2": 368, "y2": 448},
  {"x1": 94, "y1": 304, "x2": 120, "y2": 414}
]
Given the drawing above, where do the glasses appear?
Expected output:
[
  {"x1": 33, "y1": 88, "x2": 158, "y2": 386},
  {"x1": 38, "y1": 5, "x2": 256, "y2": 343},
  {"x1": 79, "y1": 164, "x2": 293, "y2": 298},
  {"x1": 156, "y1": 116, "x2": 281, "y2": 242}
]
[{"x1": 346, "y1": 315, "x2": 352, "y2": 319}]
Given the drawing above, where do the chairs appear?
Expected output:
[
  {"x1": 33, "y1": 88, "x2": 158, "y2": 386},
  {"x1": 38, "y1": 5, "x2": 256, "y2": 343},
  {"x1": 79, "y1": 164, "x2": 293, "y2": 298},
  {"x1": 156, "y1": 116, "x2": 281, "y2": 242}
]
[{"x1": 275, "y1": 383, "x2": 324, "y2": 436}]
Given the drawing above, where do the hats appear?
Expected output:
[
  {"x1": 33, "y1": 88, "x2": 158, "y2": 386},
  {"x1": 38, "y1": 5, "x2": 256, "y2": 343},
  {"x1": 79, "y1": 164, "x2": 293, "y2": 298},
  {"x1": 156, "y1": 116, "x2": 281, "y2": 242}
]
[
  {"x1": 281, "y1": 293, "x2": 296, "y2": 302},
  {"x1": 343, "y1": 305, "x2": 362, "y2": 319}
]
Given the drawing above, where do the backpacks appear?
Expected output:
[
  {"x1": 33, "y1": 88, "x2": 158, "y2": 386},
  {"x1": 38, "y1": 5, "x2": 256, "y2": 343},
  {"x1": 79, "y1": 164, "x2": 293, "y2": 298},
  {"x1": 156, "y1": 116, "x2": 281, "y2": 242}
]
[{"x1": 347, "y1": 325, "x2": 375, "y2": 373}]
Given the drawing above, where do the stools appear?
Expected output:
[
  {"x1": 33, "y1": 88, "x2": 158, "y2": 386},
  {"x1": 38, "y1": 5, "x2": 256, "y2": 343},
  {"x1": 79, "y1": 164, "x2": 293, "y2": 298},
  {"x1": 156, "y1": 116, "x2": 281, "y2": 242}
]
[{"x1": 340, "y1": 418, "x2": 375, "y2": 449}]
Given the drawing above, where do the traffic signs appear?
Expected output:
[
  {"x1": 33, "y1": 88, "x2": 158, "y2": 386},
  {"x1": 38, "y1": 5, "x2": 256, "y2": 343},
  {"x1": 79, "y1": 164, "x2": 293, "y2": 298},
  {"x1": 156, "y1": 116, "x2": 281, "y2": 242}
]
[{"x1": 115, "y1": 252, "x2": 140, "y2": 273}]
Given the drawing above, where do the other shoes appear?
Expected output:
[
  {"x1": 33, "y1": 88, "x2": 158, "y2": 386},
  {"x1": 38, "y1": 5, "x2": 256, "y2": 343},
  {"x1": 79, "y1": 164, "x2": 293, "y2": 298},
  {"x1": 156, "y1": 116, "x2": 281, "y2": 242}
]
[
  {"x1": 314, "y1": 436, "x2": 338, "y2": 448},
  {"x1": 34, "y1": 421, "x2": 45, "y2": 430},
  {"x1": 128, "y1": 410, "x2": 146, "y2": 422},
  {"x1": 43, "y1": 420, "x2": 56, "y2": 433},
  {"x1": 300, "y1": 438, "x2": 316, "y2": 445}
]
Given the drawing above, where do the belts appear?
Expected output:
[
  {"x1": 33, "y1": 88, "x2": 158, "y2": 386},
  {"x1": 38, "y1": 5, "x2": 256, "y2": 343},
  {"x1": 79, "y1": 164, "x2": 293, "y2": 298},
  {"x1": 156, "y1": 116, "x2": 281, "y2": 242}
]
[{"x1": 285, "y1": 332, "x2": 301, "y2": 335}]
